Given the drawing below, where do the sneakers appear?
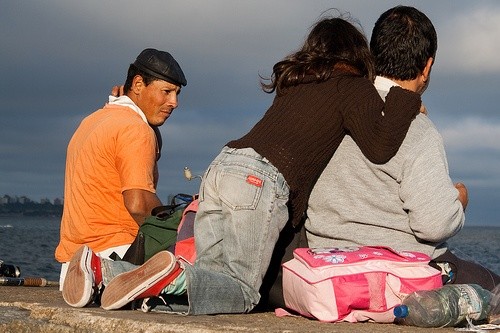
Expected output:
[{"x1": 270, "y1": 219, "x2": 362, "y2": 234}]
[
  {"x1": 100, "y1": 250, "x2": 184, "y2": 310},
  {"x1": 62, "y1": 245, "x2": 103, "y2": 308}
]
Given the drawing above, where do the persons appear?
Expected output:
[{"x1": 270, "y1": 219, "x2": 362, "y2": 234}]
[
  {"x1": 304, "y1": 6, "x2": 500, "y2": 291},
  {"x1": 63, "y1": 17, "x2": 428, "y2": 317},
  {"x1": 54, "y1": 48, "x2": 187, "y2": 292}
]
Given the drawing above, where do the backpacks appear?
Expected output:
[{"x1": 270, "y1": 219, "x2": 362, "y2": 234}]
[
  {"x1": 275, "y1": 247, "x2": 454, "y2": 324},
  {"x1": 109, "y1": 194, "x2": 193, "y2": 266},
  {"x1": 175, "y1": 193, "x2": 199, "y2": 266}
]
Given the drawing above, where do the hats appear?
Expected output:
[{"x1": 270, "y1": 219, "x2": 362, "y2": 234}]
[{"x1": 134, "y1": 49, "x2": 187, "y2": 88}]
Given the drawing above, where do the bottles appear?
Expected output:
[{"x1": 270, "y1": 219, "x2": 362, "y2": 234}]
[{"x1": 393, "y1": 282, "x2": 491, "y2": 328}]
